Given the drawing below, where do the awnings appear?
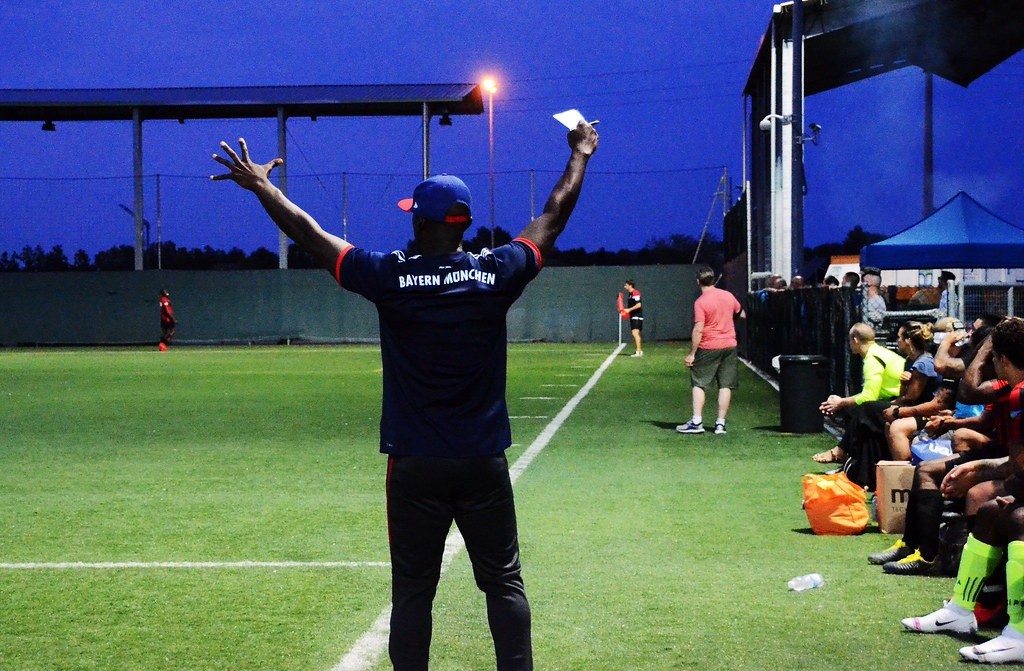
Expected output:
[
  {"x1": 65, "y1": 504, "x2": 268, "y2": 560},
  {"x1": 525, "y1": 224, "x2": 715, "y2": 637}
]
[{"x1": 744, "y1": 0, "x2": 1024, "y2": 98}]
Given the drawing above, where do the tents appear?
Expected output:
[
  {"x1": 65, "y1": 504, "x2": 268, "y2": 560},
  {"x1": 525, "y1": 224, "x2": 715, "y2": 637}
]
[{"x1": 859, "y1": 191, "x2": 1024, "y2": 326}]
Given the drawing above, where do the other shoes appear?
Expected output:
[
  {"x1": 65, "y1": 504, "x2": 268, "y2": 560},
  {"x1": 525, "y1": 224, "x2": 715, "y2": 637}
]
[{"x1": 159, "y1": 342, "x2": 167, "y2": 351}]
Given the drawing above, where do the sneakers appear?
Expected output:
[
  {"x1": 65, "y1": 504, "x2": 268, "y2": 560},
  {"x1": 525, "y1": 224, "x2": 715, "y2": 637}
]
[
  {"x1": 630, "y1": 350, "x2": 644, "y2": 358},
  {"x1": 902, "y1": 599, "x2": 978, "y2": 636},
  {"x1": 868, "y1": 539, "x2": 914, "y2": 565},
  {"x1": 676, "y1": 419, "x2": 705, "y2": 433},
  {"x1": 959, "y1": 626, "x2": 1024, "y2": 664},
  {"x1": 883, "y1": 548, "x2": 946, "y2": 575},
  {"x1": 714, "y1": 420, "x2": 727, "y2": 435},
  {"x1": 974, "y1": 602, "x2": 1007, "y2": 623}
]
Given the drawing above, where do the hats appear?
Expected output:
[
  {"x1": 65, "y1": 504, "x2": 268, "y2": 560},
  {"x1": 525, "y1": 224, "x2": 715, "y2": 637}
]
[{"x1": 397, "y1": 173, "x2": 472, "y2": 225}]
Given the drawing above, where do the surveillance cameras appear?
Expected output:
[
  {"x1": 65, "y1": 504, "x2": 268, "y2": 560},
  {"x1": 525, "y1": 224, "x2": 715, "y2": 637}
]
[{"x1": 759, "y1": 120, "x2": 771, "y2": 133}]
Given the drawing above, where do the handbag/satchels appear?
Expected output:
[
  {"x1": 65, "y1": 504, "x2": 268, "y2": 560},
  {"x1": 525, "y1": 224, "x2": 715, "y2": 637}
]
[
  {"x1": 876, "y1": 461, "x2": 916, "y2": 534},
  {"x1": 801, "y1": 471, "x2": 870, "y2": 536},
  {"x1": 911, "y1": 434, "x2": 953, "y2": 465}
]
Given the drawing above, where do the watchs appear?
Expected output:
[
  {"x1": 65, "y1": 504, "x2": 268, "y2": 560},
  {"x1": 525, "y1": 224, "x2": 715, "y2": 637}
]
[{"x1": 893, "y1": 405, "x2": 901, "y2": 419}]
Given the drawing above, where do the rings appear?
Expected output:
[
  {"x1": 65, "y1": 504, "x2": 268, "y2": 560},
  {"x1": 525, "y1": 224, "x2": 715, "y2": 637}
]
[{"x1": 595, "y1": 137, "x2": 600, "y2": 142}]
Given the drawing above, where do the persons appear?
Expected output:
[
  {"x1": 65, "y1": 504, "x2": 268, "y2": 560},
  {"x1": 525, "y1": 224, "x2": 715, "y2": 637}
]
[
  {"x1": 624, "y1": 279, "x2": 644, "y2": 357},
  {"x1": 676, "y1": 266, "x2": 746, "y2": 433},
  {"x1": 159, "y1": 288, "x2": 178, "y2": 347},
  {"x1": 750, "y1": 271, "x2": 1024, "y2": 661},
  {"x1": 210, "y1": 109, "x2": 600, "y2": 671}
]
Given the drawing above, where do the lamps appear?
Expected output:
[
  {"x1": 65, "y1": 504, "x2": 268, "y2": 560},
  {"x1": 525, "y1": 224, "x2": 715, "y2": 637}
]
[
  {"x1": 438, "y1": 113, "x2": 452, "y2": 126},
  {"x1": 41, "y1": 118, "x2": 56, "y2": 132}
]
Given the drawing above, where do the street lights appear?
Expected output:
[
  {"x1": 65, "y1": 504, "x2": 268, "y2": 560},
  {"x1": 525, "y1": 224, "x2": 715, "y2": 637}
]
[{"x1": 483, "y1": 78, "x2": 494, "y2": 249}]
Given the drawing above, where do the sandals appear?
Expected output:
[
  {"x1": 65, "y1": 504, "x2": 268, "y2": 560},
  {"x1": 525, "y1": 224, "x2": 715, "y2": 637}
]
[{"x1": 813, "y1": 449, "x2": 847, "y2": 463}]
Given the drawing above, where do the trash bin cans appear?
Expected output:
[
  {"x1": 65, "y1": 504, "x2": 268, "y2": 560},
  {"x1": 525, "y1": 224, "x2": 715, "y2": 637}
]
[{"x1": 779, "y1": 355, "x2": 829, "y2": 433}]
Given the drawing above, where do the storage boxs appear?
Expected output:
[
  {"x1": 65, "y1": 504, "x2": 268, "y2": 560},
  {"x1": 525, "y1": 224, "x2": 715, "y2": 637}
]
[{"x1": 875, "y1": 461, "x2": 917, "y2": 535}]
